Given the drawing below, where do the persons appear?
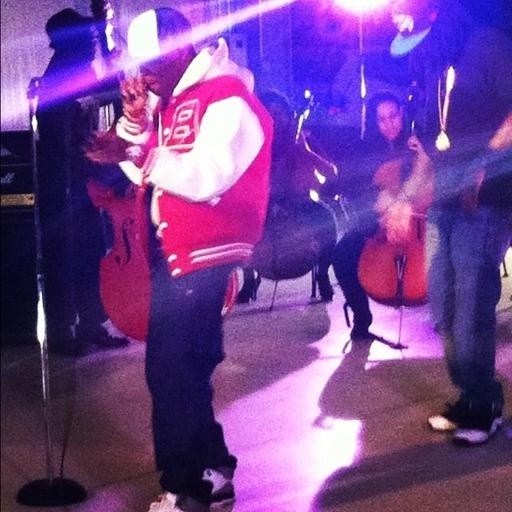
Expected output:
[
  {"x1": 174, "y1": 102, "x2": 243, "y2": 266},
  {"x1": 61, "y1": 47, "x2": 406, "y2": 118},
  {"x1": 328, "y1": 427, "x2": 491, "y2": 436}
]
[
  {"x1": 391, "y1": 1, "x2": 511, "y2": 443},
  {"x1": 334, "y1": 90, "x2": 438, "y2": 340},
  {"x1": 80, "y1": 8, "x2": 273, "y2": 512},
  {"x1": 35, "y1": 8, "x2": 134, "y2": 352},
  {"x1": 237, "y1": 89, "x2": 337, "y2": 301}
]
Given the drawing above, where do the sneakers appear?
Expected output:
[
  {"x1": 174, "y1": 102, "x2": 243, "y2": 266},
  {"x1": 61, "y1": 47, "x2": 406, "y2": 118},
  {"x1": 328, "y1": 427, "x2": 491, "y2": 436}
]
[
  {"x1": 426, "y1": 402, "x2": 505, "y2": 445},
  {"x1": 47, "y1": 327, "x2": 133, "y2": 356},
  {"x1": 145, "y1": 466, "x2": 236, "y2": 512}
]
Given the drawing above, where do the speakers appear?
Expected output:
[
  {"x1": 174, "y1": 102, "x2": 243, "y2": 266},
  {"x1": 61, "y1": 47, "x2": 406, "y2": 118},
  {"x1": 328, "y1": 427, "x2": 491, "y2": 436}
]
[{"x1": 0, "y1": 129, "x2": 39, "y2": 347}]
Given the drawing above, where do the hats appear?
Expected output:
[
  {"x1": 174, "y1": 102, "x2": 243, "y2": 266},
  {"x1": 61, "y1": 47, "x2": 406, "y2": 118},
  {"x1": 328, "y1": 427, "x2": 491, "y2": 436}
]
[
  {"x1": 388, "y1": 0, "x2": 446, "y2": 59},
  {"x1": 45, "y1": 9, "x2": 93, "y2": 48},
  {"x1": 123, "y1": 6, "x2": 192, "y2": 73}
]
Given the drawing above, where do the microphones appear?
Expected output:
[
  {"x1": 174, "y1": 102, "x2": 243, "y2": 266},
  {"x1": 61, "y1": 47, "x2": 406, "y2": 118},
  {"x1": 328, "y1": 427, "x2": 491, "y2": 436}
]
[{"x1": 75, "y1": 88, "x2": 121, "y2": 112}]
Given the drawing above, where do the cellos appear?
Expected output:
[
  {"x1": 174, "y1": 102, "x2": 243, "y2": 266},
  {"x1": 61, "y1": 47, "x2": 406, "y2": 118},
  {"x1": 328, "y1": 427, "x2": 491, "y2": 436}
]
[
  {"x1": 248, "y1": 89, "x2": 319, "y2": 280},
  {"x1": 84, "y1": 0, "x2": 236, "y2": 343},
  {"x1": 356, "y1": 86, "x2": 431, "y2": 308}
]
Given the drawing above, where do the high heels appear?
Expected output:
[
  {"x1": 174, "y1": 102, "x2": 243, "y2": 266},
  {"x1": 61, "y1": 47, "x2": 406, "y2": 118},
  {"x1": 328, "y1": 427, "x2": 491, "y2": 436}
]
[
  {"x1": 237, "y1": 269, "x2": 261, "y2": 304},
  {"x1": 344, "y1": 298, "x2": 372, "y2": 339},
  {"x1": 317, "y1": 272, "x2": 334, "y2": 300}
]
